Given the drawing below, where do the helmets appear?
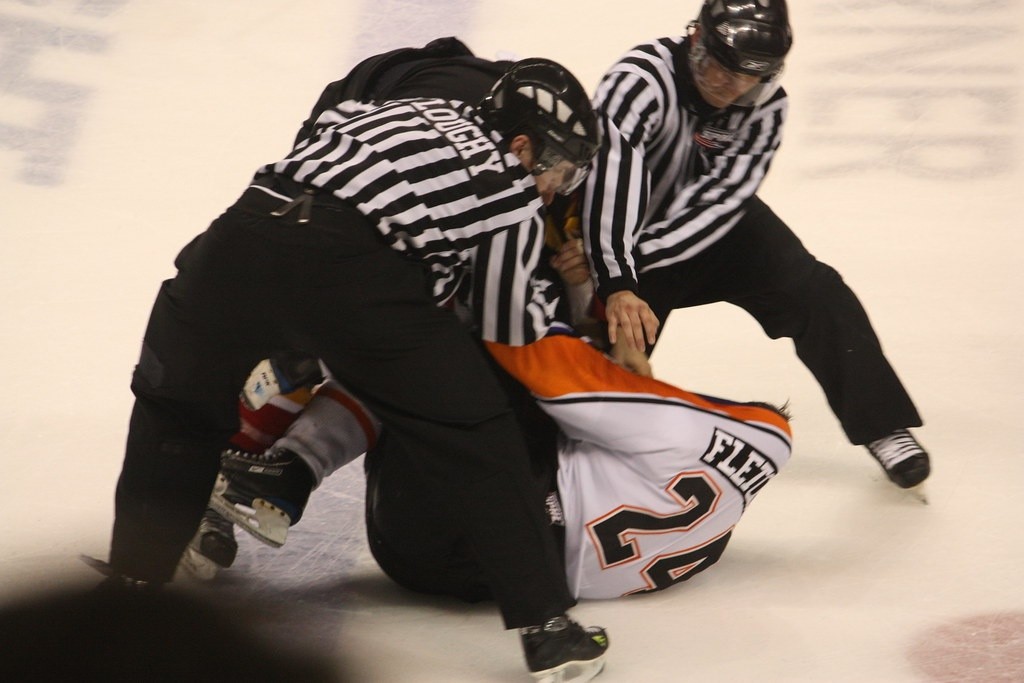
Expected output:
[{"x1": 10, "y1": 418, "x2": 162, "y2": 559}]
[
  {"x1": 481, "y1": 58, "x2": 602, "y2": 167},
  {"x1": 699, "y1": 0, "x2": 792, "y2": 79}
]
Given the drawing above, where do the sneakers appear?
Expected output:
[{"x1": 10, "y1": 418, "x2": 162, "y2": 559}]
[
  {"x1": 520, "y1": 620, "x2": 607, "y2": 683},
  {"x1": 867, "y1": 428, "x2": 931, "y2": 506},
  {"x1": 181, "y1": 508, "x2": 238, "y2": 581},
  {"x1": 205, "y1": 450, "x2": 315, "y2": 548}
]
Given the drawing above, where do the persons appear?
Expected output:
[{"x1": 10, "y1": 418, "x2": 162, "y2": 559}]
[
  {"x1": 109, "y1": 55, "x2": 612, "y2": 672},
  {"x1": 221, "y1": 224, "x2": 795, "y2": 606},
  {"x1": 550, "y1": 0, "x2": 929, "y2": 490},
  {"x1": 176, "y1": 34, "x2": 656, "y2": 578}
]
